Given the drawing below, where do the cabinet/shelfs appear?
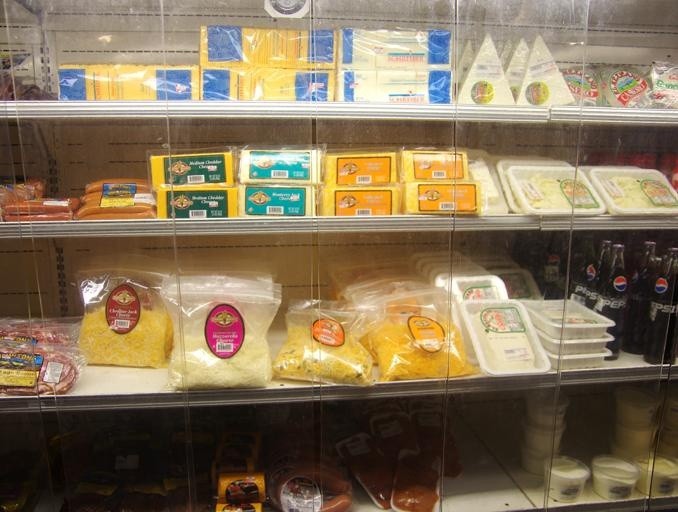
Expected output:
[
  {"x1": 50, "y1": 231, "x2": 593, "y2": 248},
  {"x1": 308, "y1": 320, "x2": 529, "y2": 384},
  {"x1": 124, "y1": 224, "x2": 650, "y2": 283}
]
[{"x1": 0, "y1": 3, "x2": 677, "y2": 512}]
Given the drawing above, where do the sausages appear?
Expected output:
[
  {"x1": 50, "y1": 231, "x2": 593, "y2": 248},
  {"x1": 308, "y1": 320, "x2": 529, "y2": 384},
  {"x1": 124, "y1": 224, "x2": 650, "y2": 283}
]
[
  {"x1": 277, "y1": 467, "x2": 353, "y2": 512},
  {"x1": 2, "y1": 178, "x2": 156, "y2": 223}
]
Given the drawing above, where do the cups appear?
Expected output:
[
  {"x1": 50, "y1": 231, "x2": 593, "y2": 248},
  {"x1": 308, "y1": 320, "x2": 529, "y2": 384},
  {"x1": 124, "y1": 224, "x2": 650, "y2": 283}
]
[{"x1": 517, "y1": 386, "x2": 678, "y2": 502}]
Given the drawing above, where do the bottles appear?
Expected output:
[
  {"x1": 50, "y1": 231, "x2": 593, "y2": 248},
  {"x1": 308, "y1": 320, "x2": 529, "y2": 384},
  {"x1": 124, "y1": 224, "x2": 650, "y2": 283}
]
[{"x1": 568, "y1": 231, "x2": 676, "y2": 370}]
